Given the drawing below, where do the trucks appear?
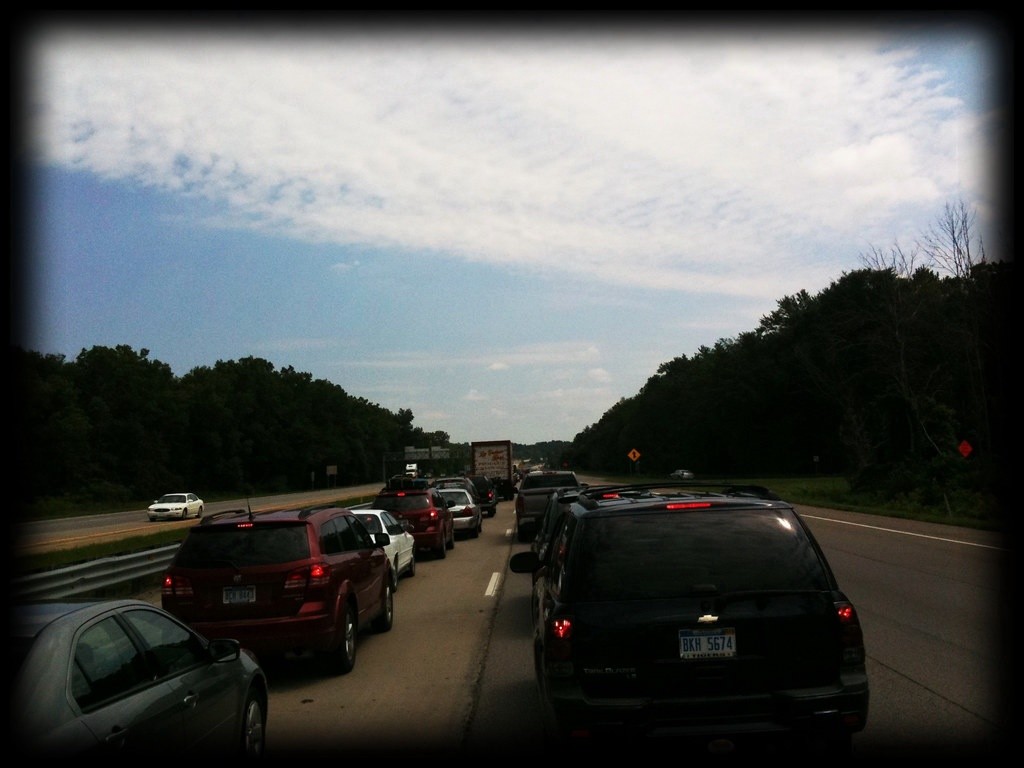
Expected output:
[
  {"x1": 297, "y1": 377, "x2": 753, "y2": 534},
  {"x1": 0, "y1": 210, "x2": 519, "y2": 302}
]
[
  {"x1": 405, "y1": 463, "x2": 418, "y2": 478},
  {"x1": 471, "y1": 441, "x2": 514, "y2": 500}
]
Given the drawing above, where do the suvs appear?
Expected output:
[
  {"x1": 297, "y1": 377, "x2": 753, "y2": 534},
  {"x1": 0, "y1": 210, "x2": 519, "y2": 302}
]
[
  {"x1": 514, "y1": 470, "x2": 588, "y2": 541},
  {"x1": 509, "y1": 482, "x2": 870, "y2": 736},
  {"x1": 162, "y1": 505, "x2": 394, "y2": 675},
  {"x1": 372, "y1": 487, "x2": 456, "y2": 559},
  {"x1": 429, "y1": 475, "x2": 481, "y2": 510},
  {"x1": 464, "y1": 476, "x2": 497, "y2": 517}
]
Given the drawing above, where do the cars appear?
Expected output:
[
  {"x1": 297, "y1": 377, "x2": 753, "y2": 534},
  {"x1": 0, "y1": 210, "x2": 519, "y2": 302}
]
[
  {"x1": 390, "y1": 475, "x2": 404, "y2": 481},
  {"x1": 147, "y1": 492, "x2": 205, "y2": 521},
  {"x1": 670, "y1": 470, "x2": 694, "y2": 480},
  {"x1": 416, "y1": 477, "x2": 428, "y2": 485},
  {"x1": 514, "y1": 468, "x2": 529, "y2": 482},
  {"x1": 19, "y1": 598, "x2": 268, "y2": 755},
  {"x1": 347, "y1": 510, "x2": 416, "y2": 593},
  {"x1": 437, "y1": 489, "x2": 483, "y2": 537}
]
